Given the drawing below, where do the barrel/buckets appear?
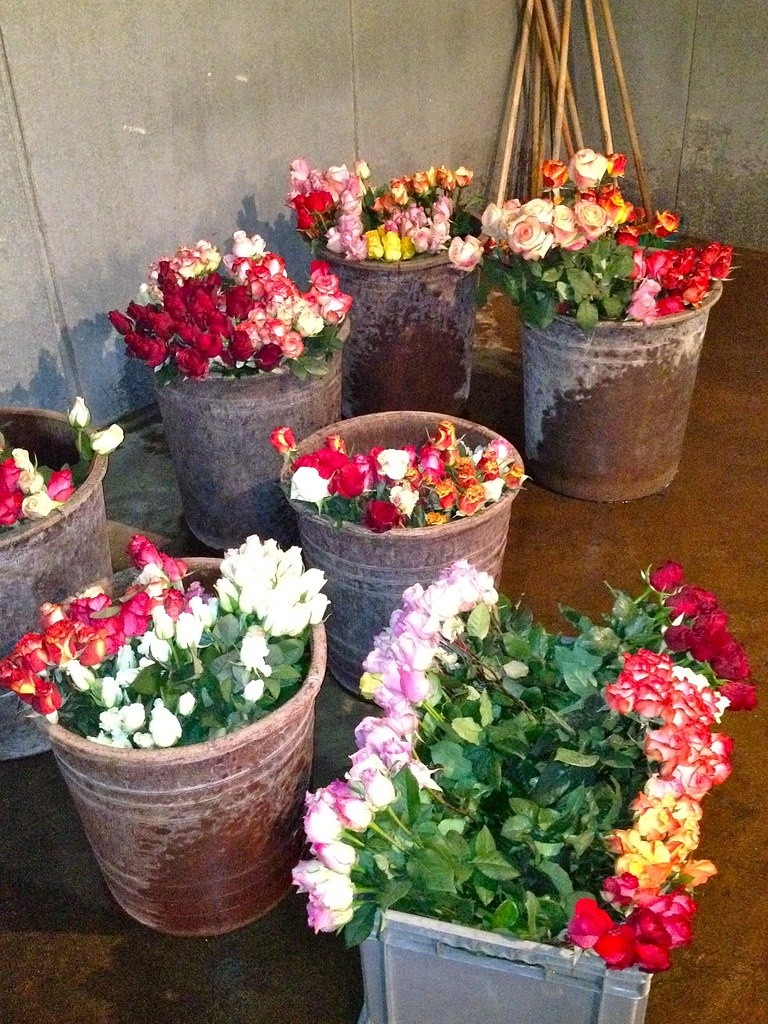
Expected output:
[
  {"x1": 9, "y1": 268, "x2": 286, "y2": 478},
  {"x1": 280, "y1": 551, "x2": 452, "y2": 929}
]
[
  {"x1": 37, "y1": 558, "x2": 327, "y2": 933},
  {"x1": 316, "y1": 245, "x2": 476, "y2": 415},
  {"x1": 281, "y1": 411, "x2": 521, "y2": 697},
  {"x1": 523, "y1": 278, "x2": 722, "y2": 498},
  {"x1": 150, "y1": 319, "x2": 344, "y2": 548},
  {"x1": 0, "y1": 405, "x2": 111, "y2": 761}
]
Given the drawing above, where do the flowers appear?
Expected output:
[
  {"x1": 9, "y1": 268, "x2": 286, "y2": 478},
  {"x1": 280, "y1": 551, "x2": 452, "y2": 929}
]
[
  {"x1": 267, "y1": 421, "x2": 534, "y2": 535},
  {"x1": 0, "y1": 532, "x2": 334, "y2": 748},
  {"x1": 287, "y1": 157, "x2": 485, "y2": 261},
  {"x1": 290, "y1": 555, "x2": 757, "y2": 975},
  {"x1": 0, "y1": 393, "x2": 126, "y2": 531},
  {"x1": 447, "y1": 148, "x2": 736, "y2": 333},
  {"x1": 106, "y1": 228, "x2": 355, "y2": 386}
]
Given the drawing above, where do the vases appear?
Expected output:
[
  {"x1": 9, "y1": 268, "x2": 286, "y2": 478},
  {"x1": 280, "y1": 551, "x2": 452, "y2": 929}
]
[
  {"x1": 357, "y1": 907, "x2": 652, "y2": 1024},
  {"x1": 280, "y1": 411, "x2": 525, "y2": 698},
  {"x1": 316, "y1": 243, "x2": 481, "y2": 420},
  {"x1": 154, "y1": 318, "x2": 352, "y2": 550},
  {"x1": 37, "y1": 556, "x2": 327, "y2": 937},
  {"x1": 0, "y1": 405, "x2": 116, "y2": 761},
  {"x1": 523, "y1": 280, "x2": 724, "y2": 502}
]
